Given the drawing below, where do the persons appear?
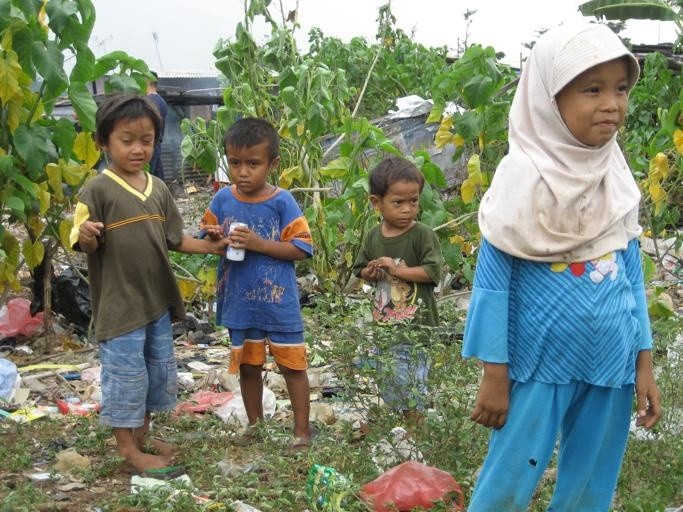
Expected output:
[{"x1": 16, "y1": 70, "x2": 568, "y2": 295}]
[
  {"x1": 457, "y1": 17, "x2": 664, "y2": 512},
  {"x1": 137, "y1": 69, "x2": 168, "y2": 176},
  {"x1": 348, "y1": 158, "x2": 443, "y2": 416},
  {"x1": 68, "y1": 93, "x2": 231, "y2": 475},
  {"x1": 198, "y1": 118, "x2": 316, "y2": 455}
]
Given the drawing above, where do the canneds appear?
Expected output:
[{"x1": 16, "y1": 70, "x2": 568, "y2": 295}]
[{"x1": 226, "y1": 221, "x2": 249, "y2": 262}]
